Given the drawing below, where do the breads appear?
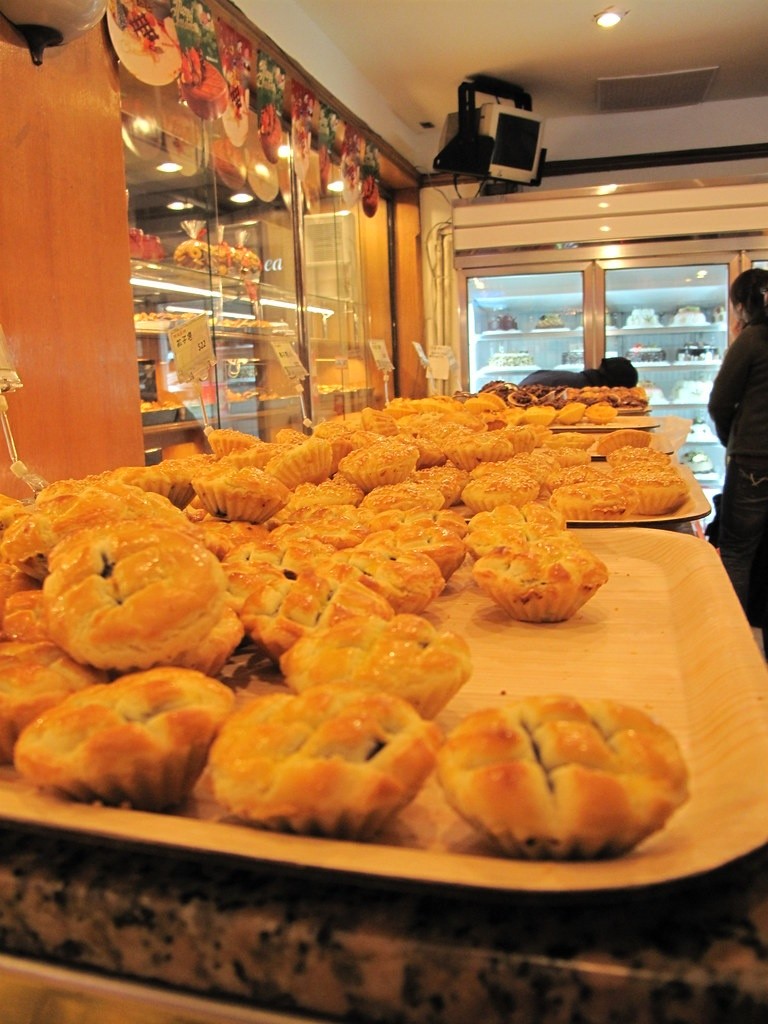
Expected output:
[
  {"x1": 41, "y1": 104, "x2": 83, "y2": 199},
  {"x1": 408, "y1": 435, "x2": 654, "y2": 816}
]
[{"x1": 0, "y1": 381, "x2": 690, "y2": 854}]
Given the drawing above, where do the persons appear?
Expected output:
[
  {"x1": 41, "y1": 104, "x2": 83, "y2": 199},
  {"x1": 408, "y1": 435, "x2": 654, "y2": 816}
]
[
  {"x1": 518, "y1": 357, "x2": 639, "y2": 389},
  {"x1": 707, "y1": 268, "x2": 768, "y2": 664}
]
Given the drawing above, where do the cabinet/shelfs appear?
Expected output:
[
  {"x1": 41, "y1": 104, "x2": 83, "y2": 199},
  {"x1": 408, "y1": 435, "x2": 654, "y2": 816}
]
[{"x1": 130, "y1": 257, "x2": 384, "y2": 450}]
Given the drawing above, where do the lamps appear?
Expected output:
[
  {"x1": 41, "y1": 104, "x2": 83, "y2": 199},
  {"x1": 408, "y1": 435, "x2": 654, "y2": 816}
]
[{"x1": 0, "y1": 0, "x2": 108, "y2": 66}]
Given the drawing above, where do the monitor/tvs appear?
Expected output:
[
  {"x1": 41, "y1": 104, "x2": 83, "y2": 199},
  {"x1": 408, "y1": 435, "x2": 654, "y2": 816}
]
[{"x1": 437, "y1": 101, "x2": 543, "y2": 184}]
